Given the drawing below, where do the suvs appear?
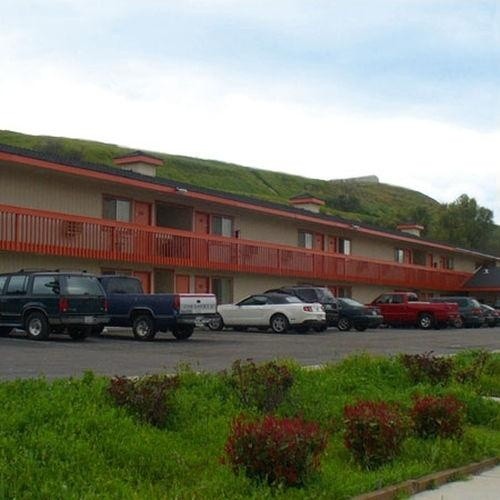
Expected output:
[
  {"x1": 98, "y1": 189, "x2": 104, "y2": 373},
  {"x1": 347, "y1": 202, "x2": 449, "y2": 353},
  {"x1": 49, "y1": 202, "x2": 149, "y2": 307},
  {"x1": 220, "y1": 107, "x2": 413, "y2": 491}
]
[
  {"x1": 0, "y1": 269, "x2": 112, "y2": 340},
  {"x1": 430, "y1": 296, "x2": 486, "y2": 328},
  {"x1": 266, "y1": 286, "x2": 343, "y2": 335}
]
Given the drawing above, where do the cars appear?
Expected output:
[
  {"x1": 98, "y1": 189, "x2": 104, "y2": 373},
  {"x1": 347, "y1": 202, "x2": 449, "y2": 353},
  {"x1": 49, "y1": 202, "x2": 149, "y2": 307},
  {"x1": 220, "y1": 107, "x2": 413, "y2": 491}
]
[
  {"x1": 203, "y1": 293, "x2": 327, "y2": 334},
  {"x1": 327, "y1": 298, "x2": 383, "y2": 332},
  {"x1": 473, "y1": 304, "x2": 500, "y2": 329}
]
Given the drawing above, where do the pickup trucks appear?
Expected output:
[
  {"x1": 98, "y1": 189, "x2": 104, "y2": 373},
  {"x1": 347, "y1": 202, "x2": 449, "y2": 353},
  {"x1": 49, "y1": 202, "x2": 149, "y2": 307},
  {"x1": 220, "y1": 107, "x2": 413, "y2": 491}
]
[
  {"x1": 89, "y1": 275, "x2": 217, "y2": 341},
  {"x1": 364, "y1": 292, "x2": 458, "y2": 330}
]
